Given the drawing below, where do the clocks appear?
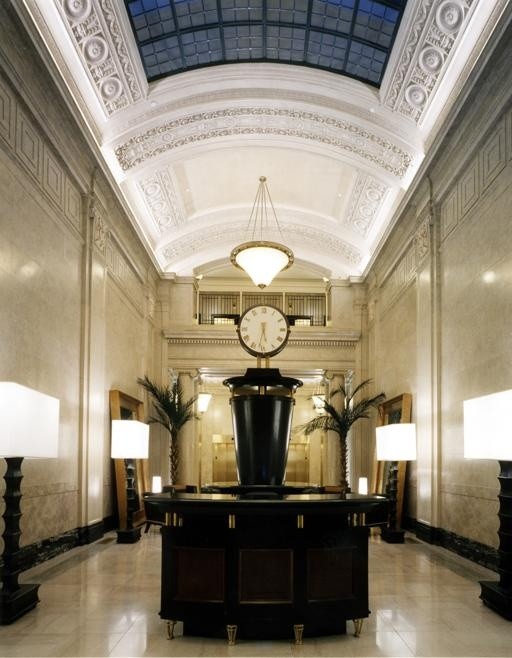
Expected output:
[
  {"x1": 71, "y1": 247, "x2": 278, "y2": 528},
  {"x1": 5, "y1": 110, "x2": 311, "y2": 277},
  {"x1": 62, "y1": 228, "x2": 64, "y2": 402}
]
[{"x1": 237, "y1": 305, "x2": 291, "y2": 356}]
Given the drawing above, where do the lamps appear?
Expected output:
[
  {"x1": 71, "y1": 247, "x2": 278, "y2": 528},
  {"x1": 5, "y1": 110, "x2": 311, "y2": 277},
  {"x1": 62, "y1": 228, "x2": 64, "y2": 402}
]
[
  {"x1": 372, "y1": 422, "x2": 418, "y2": 544},
  {"x1": 0, "y1": 381, "x2": 61, "y2": 624},
  {"x1": 109, "y1": 418, "x2": 150, "y2": 545},
  {"x1": 462, "y1": 389, "x2": 512, "y2": 624},
  {"x1": 228, "y1": 175, "x2": 294, "y2": 289}
]
